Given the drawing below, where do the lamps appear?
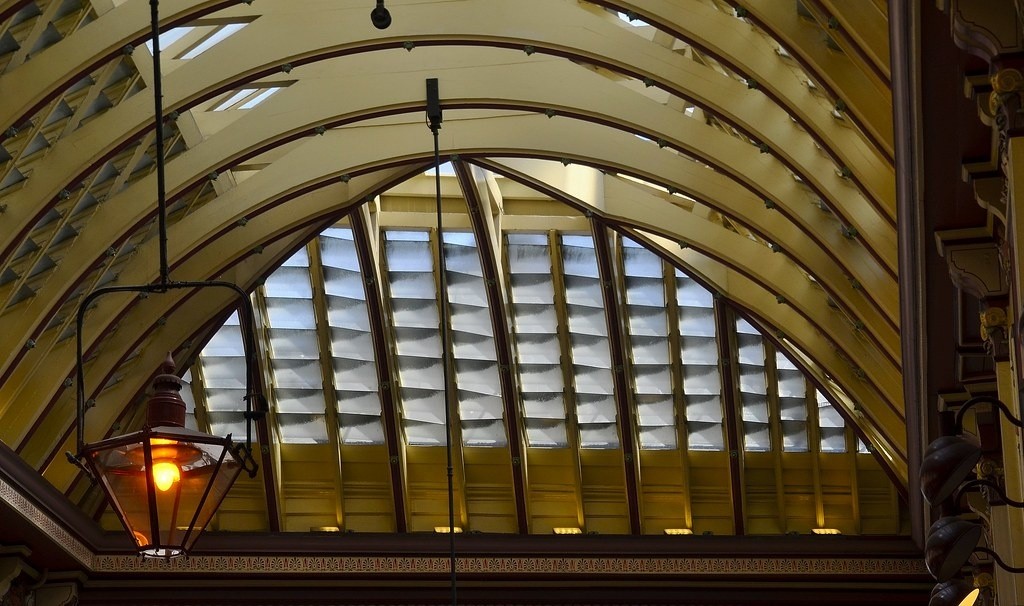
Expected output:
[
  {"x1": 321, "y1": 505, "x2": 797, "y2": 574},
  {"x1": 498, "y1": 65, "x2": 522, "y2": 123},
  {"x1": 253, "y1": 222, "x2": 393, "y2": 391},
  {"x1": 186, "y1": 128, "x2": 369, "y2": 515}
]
[
  {"x1": 66, "y1": 0, "x2": 266, "y2": 557},
  {"x1": 910, "y1": 394, "x2": 1024, "y2": 605}
]
